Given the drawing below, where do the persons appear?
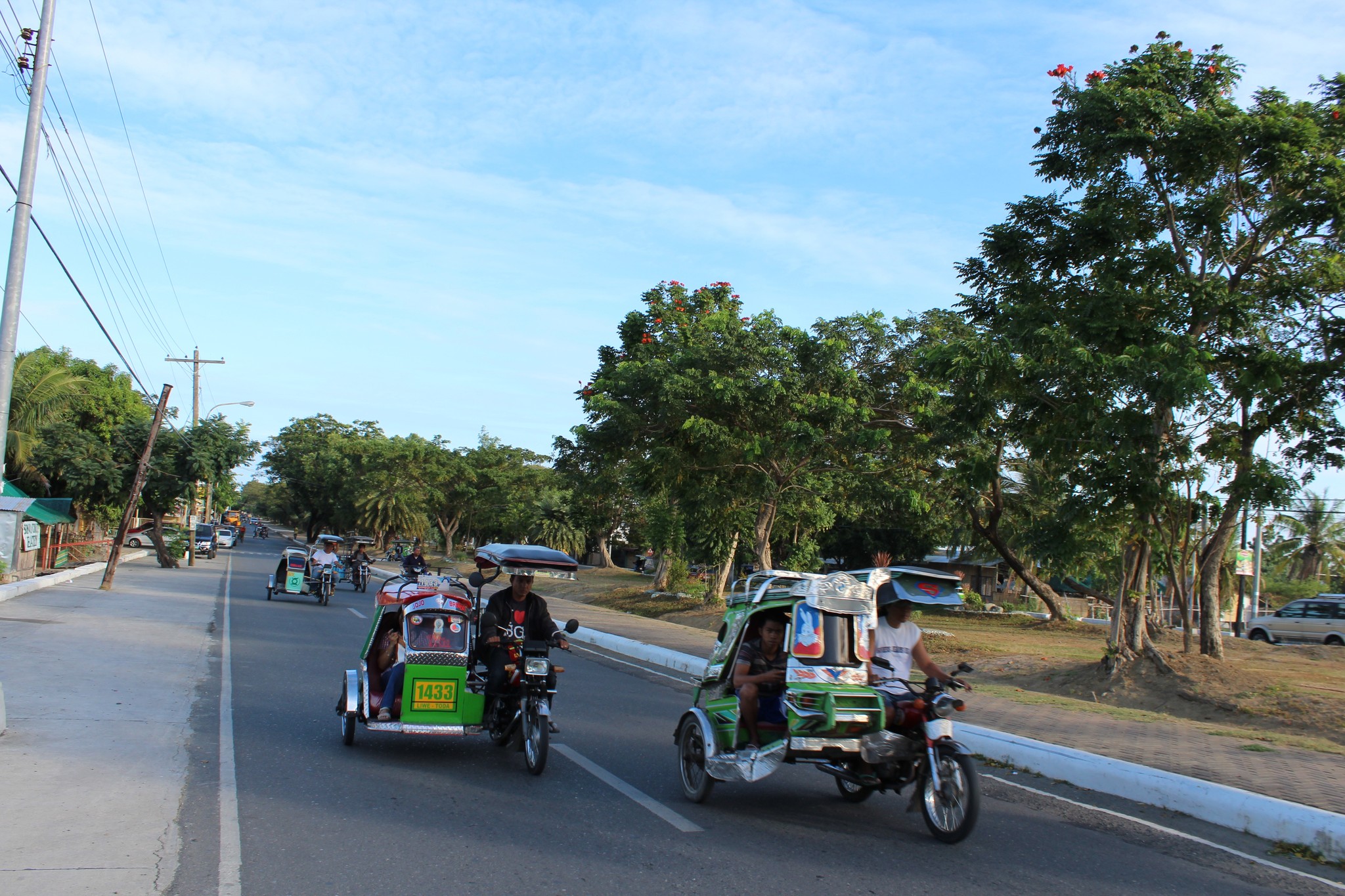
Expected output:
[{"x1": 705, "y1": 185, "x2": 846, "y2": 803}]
[
  {"x1": 293, "y1": 526, "x2": 298, "y2": 539},
  {"x1": 403, "y1": 546, "x2": 432, "y2": 581},
  {"x1": 258, "y1": 522, "x2": 261, "y2": 525},
  {"x1": 348, "y1": 544, "x2": 353, "y2": 554},
  {"x1": 259, "y1": 527, "x2": 269, "y2": 538},
  {"x1": 867, "y1": 598, "x2": 972, "y2": 731},
  {"x1": 239, "y1": 526, "x2": 246, "y2": 543},
  {"x1": 314, "y1": 542, "x2": 339, "y2": 553},
  {"x1": 464, "y1": 539, "x2": 467, "y2": 547},
  {"x1": 309, "y1": 543, "x2": 343, "y2": 596},
  {"x1": 349, "y1": 544, "x2": 374, "y2": 584},
  {"x1": 230, "y1": 521, "x2": 236, "y2": 526},
  {"x1": 474, "y1": 574, "x2": 569, "y2": 733},
  {"x1": 733, "y1": 613, "x2": 788, "y2": 749},
  {"x1": 438, "y1": 578, "x2": 449, "y2": 590},
  {"x1": 633, "y1": 557, "x2": 642, "y2": 571},
  {"x1": 377, "y1": 607, "x2": 431, "y2": 722},
  {"x1": 394, "y1": 544, "x2": 405, "y2": 561}
]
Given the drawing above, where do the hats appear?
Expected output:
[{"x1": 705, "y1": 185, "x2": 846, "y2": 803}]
[{"x1": 878, "y1": 591, "x2": 900, "y2": 608}]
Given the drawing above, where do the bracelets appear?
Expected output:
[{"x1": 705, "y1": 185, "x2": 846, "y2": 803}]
[{"x1": 386, "y1": 651, "x2": 390, "y2": 656}]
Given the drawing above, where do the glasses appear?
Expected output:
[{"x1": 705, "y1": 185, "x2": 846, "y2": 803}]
[{"x1": 890, "y1": 601, "x2": 912, "y2": 608}]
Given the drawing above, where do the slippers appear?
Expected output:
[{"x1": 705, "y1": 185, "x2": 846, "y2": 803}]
[{"x1": 853, "y1": 770, "x2": 881, "y2": 785}]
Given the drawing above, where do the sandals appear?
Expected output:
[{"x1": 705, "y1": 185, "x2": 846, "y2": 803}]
[
  {"x1": 548, "y1": 721, "x2": 560, "y2": 733},
  {"x1": 483, "y1": 714, "x2": 494, "y2": 729},
  {"x1": 744, "y1": 744, "x2": 760, "y2": 752},
  {"x1": 377, "y1": 706, "x2": 391, "y2": 722}
]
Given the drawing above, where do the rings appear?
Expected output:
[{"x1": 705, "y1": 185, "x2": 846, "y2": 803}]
[{"x1": 565, "y1": 646, "x2": 566, "y2": 647}]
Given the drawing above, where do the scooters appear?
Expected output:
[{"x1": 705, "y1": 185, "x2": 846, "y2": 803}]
[{"x1": 402, "y1": 562, "x2": 432, "y2": 581}]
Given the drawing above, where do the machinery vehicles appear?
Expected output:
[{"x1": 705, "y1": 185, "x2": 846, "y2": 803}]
[{"x1": 224, "y1": 510, "x2": 241, "y2": 526}]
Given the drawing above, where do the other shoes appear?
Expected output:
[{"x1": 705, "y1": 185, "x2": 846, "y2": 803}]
[
  {"x1": 331, "y1": 587, "x2": 335, "y2": 592},
  {"x1": 309, "y1": 590, "x2": 315, "y2": 594},
  {"x1": 273, "y1": 589, "x2": 279, "y2": 595}
]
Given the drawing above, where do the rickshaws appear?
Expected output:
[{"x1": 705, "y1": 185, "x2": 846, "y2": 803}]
[
  {"x1": 265, "y1": 534, "x2": 346, "y2": 606},
  {"x1": 673, "y1": 565, "x2": 982, "y2": 844},
  {"x1": 253, "y1": 525, "x2": 269, "y2": 540},
  {"x1": 334, "y1": 543, "x2": 580, "y2": 776},
  {"x1": 380, "y1": 539, "x2": 414, "y2": 561},
  {"x1": 332, "y1": 536, "x2": 376, "y2": 593}
]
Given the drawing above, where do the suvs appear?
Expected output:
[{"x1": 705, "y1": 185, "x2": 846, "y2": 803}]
[{"x1": 179, "y1": 523, "x2": 217, "y2": 560}]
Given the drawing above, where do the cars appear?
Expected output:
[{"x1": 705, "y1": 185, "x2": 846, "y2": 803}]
[
  {"x1": 123, "y1": 526, "x2": 181, "y2": 548},
  {"x1": 210, "y1": 520, "x2": 240, "y2": 549},
  {"x1": 127, "y1": 521, "x2": 185, "y2": 534},
  {"x1": 249, "y1": 518, "x2": 259, "y2": 525},
  {"x1": 1247, "y1": 596, "x2": 1345, "y2": 646}
]
